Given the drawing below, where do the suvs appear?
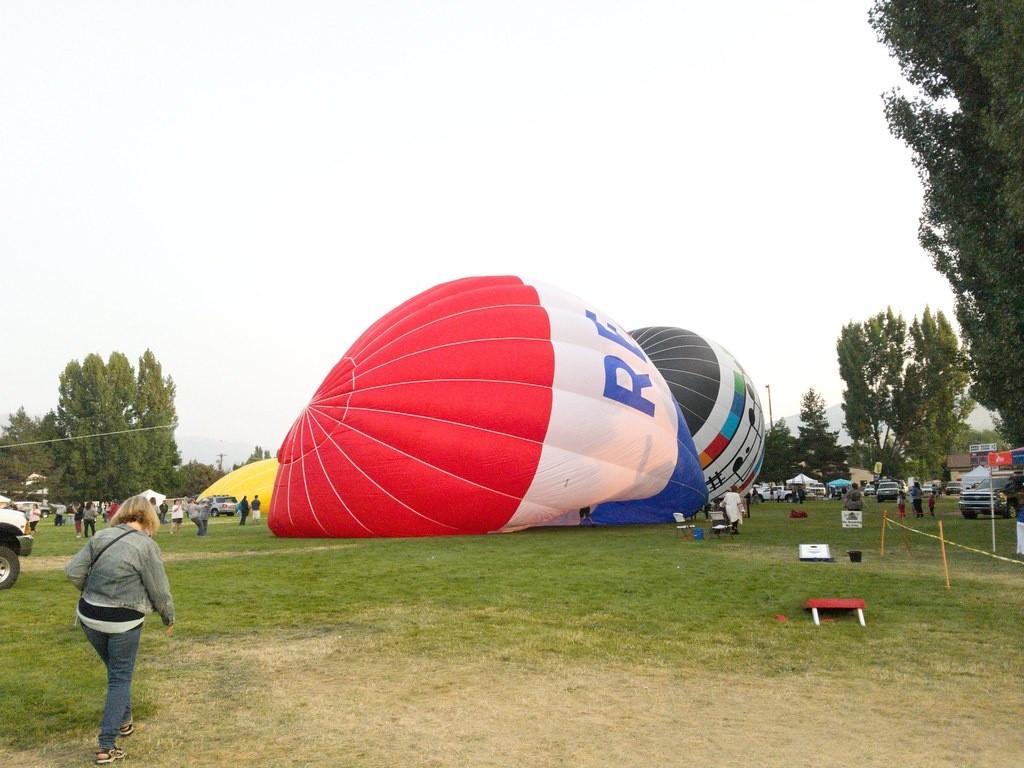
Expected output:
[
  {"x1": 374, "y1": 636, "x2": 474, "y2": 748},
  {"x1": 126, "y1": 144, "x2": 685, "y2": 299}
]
[
  {"x1": 957, "y1": 469, "x2": 1024, "y2": 520},
  {"x1": 14, "y1": 501, "x2": 52, "y2": 519},
  {"x1": 0, "y1": 507, "x2": 35, "y2": 592},
  {"x1": 876, "y1": 482, "x2": 901, "y2": 503},
  {"x1": 196, "y1": 494, "x2": 238, "y2": 517}
]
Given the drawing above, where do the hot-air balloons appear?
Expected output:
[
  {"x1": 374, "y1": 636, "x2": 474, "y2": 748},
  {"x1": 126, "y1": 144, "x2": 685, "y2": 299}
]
[
  {"x1": 194, "y1": 458, "x2": 281, "y2": 517},
  {"x1": 624, "y1": 324, "x2": 766, "y2": 509},
  {"x1": 267, "y1": 273, "x2": 709, "y2": 541}
]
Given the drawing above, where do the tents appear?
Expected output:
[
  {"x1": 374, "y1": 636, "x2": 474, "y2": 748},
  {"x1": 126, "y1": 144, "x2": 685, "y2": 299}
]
[
  {"x1": 786, "y1": 473, "x2": 818, "y2": 484},
  {"x1": 962, "y1": 465, "x2": 990, "y2": 489},
  {"x1": 989, "y1": 447, "x2": 1024, "y2": 553},
  {"x1": 141, "y1": 489, "x2": 167, "y2": 506},
  {"x1": 827, "y1": 478, "x2": 852, "y2": 487}
]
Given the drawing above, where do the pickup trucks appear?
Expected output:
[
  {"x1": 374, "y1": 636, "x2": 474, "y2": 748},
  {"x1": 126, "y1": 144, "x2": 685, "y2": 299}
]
[{"x1": 758, "y1": 484, "x2": 800, "y2": 504}]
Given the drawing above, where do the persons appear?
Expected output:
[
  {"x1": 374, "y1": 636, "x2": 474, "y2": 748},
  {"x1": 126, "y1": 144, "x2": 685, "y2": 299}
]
[
  {"x1": 910, "y1": 481, "x2": 924, "y2": 518},
  {"x1": 237, "y1": 494, "x2": 262, "y2": 526},
  {"x1": 74, "y1": 500, "x2": 97, "y2": 539},
  {"x1": 579, "y1": 506, "x2": 598, "y2": 528},
  {"x1": 159, "y1": 500, "x2": 168, "y2": 523},
  {"x1": 9, "y1": 501, "x2": 18, "y2": 511},
  {"x1": 29, "y1": 503, "x2": 41, "y2": 535},
  {"x1": 703, "y1": 487, "x2": 760, "y2": 535},
  {"x1": 185, "y1": 497, "x2": 215, "y2": 537},
  {"x1": 149, "y1": 497, "x2": 161, "y2": 514},
  {"x1": 928, "y1": 490, "x2": 936, "y2": 518},
  {"x1": 49, "y1": 501, "x2": 78, "y2": 526},
  {"x1": 932, "y1": 483, "x2": 944, "y2": 498},
  {"x1": 770, "y1": 482, "x2": 871, "y2": 512},
  {"x1": 100, "y1": 499, "x2": 124, "y2": 523},
  {"x1": 170, "y1": 498, "x2": 183, "y2": 535},
  {"x1": 897, "y1": 490, "x2": 909, "y2": 519},
  {"x1": 64, "y1": 495, "x2": 175, "y2": 763}
]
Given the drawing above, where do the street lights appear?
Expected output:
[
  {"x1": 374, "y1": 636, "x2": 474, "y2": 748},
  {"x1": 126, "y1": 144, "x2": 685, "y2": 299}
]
[{"x1": 765, "y1": 384, "x2": 773, "y2": 433}]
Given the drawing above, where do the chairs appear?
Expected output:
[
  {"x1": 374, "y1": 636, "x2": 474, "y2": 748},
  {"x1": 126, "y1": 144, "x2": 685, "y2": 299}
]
[
  {"x1": 673, "y1": 511, "x2": 697, "y2": 538},
  {"x1": 708, "y1": 511, "x2": 734, "y2": 541}
]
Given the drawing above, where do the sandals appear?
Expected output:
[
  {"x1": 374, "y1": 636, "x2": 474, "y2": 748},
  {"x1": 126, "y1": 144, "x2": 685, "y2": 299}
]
[
  {"x1": 96, "y1": 744, "x2": 125, "y2": 763},
  {"x1": 119, "y1": 711, "x2": 134, "y2": 735}
]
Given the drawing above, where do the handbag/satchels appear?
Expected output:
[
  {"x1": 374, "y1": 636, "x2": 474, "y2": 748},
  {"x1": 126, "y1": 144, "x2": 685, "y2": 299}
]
[
  {"x1": 790, "y1": 509, "x2": 808, "y2": 518},
  {"x1": 693, "y1": 528, "x2": 704, "y2": 539}
]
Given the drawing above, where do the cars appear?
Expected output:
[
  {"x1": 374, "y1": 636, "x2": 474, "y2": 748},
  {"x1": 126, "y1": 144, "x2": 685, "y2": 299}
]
[
  {"x1": 810, "y1": 483, "x2": 826, "y2": 495},
  {"x1": 922, "y1": 483, "x2": 932, "y2": 494},
  {"x1": 945, "y1": 481, "x2": 963, "y2": 496},
  {"x1": 864, "y1": 484, "x2": 877, "y2": 496}
]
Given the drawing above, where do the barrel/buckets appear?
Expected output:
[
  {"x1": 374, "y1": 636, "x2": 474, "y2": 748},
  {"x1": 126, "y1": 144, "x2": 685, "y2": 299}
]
[
  {"x1": 694, "y1": 529, "x2": 704, "y2": 540},
  {"x1": 849, "y1": 550, "x2": 861, "y2": 562}
]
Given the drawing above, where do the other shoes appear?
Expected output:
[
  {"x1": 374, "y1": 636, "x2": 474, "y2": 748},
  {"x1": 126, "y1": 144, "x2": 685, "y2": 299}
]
[
  {"x1": 732, "y1": 531, "x2": 740, "y2": 534},
  {"x1": 76, "y1": 535, "x2": 82, "y2": 538}
]
